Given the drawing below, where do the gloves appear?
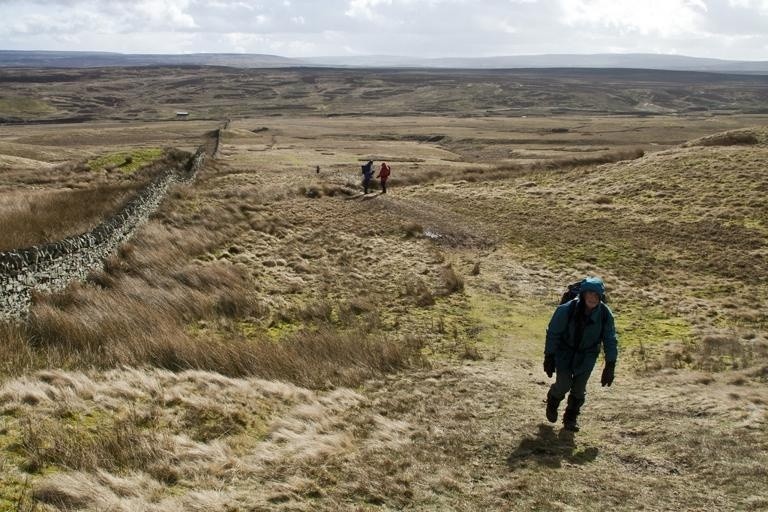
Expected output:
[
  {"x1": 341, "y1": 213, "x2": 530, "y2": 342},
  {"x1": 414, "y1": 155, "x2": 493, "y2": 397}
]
[
  {"x1": 600, "y1": 359, "x2": 615, "y2": 387},
  {"x1": 543, "y1": 353, "x2": 556, "y2": 378}
]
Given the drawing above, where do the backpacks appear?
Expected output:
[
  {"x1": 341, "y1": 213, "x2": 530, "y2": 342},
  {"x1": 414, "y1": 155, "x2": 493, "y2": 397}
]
[{"x1": 560, "y1": 281, "x2": 607, "y2": 369}]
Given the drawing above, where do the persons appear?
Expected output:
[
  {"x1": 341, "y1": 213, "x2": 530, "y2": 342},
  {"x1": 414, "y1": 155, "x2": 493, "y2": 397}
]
[
  {"x1": 362, "y1": 159, "x2": 375, "y2": 194},
  {"x1": 543, "y1": 276, "x2": 618, "y2": 433},
  {"x1": 376, "y1": 162, "x2": 389, "y2": 194}
]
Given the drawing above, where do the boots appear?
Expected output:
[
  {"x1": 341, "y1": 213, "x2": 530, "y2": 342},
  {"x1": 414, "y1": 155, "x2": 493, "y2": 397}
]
[
  {"x1": 543, "y1": 389, "x2": 564, "y2": 423},
  {"x1": 562, "y1": 394, "x2": 584, "y2": 432}
]
